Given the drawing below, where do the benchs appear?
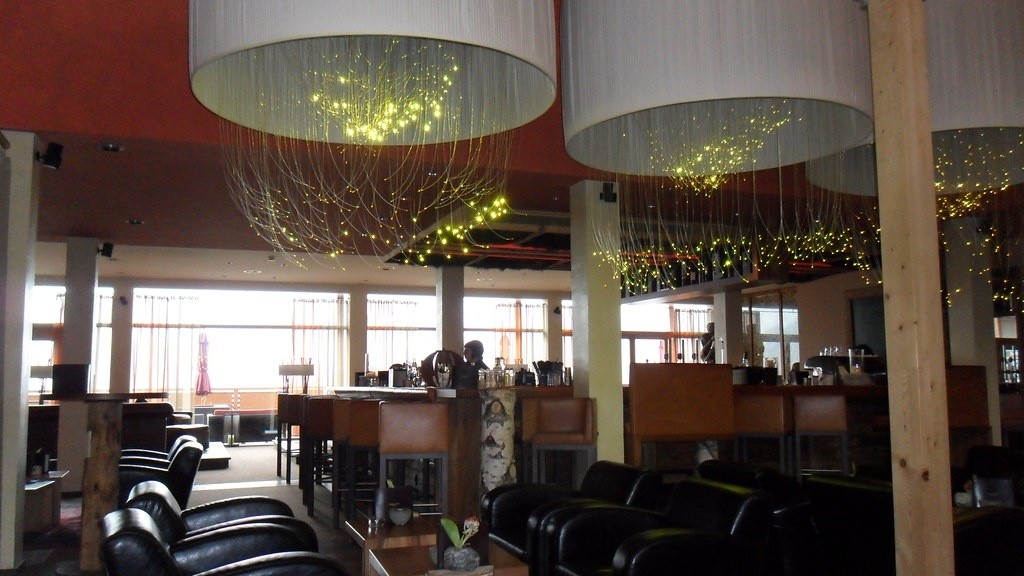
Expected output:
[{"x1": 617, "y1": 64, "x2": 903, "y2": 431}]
[
  {"x1": 165, "y1": 423, "x2": 210, "y2": 451},
  {"x1": 207, "y1": 409, "x2": 280, "y2": 447},
  {"x1": 26, "y1": 404, "x2": 173, "y2": 475}
]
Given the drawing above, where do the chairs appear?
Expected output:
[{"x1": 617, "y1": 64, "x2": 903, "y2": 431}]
[
  {"x1": 192, "y1": 402, "x2": 230, "y2": 425},
  {"x1": 98, "y1": 363, "x2": 1024, "y2": 576}
]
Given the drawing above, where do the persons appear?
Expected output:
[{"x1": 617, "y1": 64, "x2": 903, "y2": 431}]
[
  {"x1": 463, "y1": 341, "x2": 489, "y2": 373},
  {"x1": 700, "y1": 322, "x2": 715, "y2": 364},
  {"x1": 791, "y1": 362, "x2": 811, "y2": 385}
]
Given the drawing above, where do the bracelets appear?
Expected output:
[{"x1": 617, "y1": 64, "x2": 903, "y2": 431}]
[{"x1": 710, "y1": 347, "x2": 713, "y2": 350}]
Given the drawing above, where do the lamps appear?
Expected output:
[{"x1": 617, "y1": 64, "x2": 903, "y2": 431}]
[
  {"x1": 188, "y1": 1, "x2": 557, "y2": 274},
  {"x1": 560, "y1": 0, "x2": 1024, "y2": 319},
  {"x1": 96, "y1": 242, "x2": 114, "y2": 257},
  {"x1": 37, "y1": 141, "x2": 64, "y2": 169}
]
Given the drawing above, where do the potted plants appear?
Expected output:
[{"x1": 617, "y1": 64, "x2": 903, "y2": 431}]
[{"x1": 440, "y1": 516, "x2": 480, "y2": 572}]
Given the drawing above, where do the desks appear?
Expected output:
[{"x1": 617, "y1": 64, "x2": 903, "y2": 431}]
[
  {"x1": 25, "y1": 470, "x2": 71, "y2": 534},
  {"x1": 368, "y1": 538, "x2": 529, "y2": 576},
  {"x1": 341, "y1": 511, "x2": 437, "y2": 575},
  {"x1": 39, "y1": 392, "x2": 169, "y2": 404}
]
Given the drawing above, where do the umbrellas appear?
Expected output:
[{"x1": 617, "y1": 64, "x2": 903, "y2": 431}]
[{"x1": 195, "y1": 332, "x2": 212, "y2": 405}]
